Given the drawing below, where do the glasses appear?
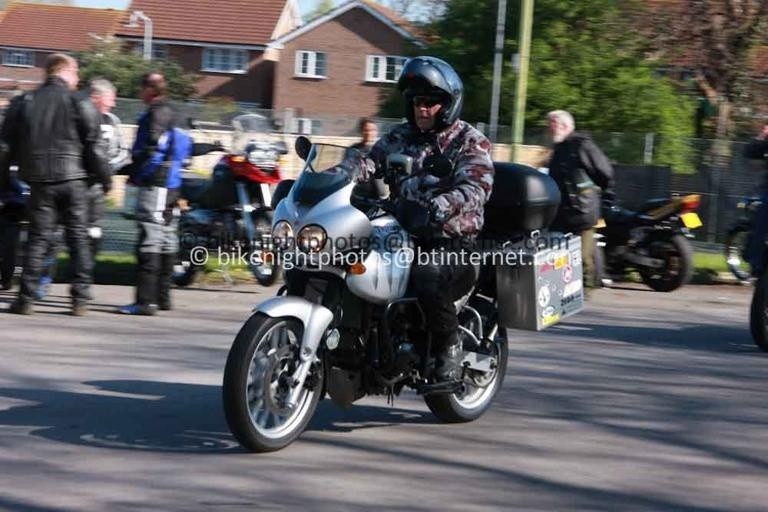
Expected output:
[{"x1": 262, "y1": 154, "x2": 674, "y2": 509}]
[{"x1": 412, "y1": 94, "x2": 441, "y2": 107}]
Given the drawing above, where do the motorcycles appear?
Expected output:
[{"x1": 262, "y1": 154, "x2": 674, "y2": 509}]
[
  {"x1": 596, "y1": 188, "x2": 702, "y2": 293},
  {"x1": 169, "y1": 141, "x2": 287, "y2": 287},
  {"x1": 223, "y1": 135, "x2": 585, "y2": 452}
]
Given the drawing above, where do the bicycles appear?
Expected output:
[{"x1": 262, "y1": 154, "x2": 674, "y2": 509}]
[{"x1": 723, "y1": 196, "x2": 757, "y2": 280}]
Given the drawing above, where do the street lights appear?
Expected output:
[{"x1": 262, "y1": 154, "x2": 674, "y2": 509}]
[{"x1": 127, "y1": 10, "x2": 152, "y2": 62}]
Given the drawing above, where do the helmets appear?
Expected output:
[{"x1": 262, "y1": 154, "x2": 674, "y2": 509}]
[{"x1": 399, "y1": 55, "x2": 464, "y2": 126}]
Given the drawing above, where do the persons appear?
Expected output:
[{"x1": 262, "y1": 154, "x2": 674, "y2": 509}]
[
  {"x1": 742, "y1": 119, "x2": 767, "y2": 163},
  {"x1": 112, "y1": 70, "x2": 187, "y2": 319},
  {"x1": 541, "y1": 106, "x2": 617, "y2": 301},
  {"x1": 31, "y1": 73, "x2": 127, "y2": 300},
  {"x1": 0, "y1": 82, "x2": 32, "y2": 294},
  {"x1": 0, "y1": 52, "x2": 104, "y2": 319},
  {"x1": 319, "y1": 54, "x2": 496, "y2": 383},
  {"x1": 339, "y1": 117, "x2": 391, "y2": 221}
]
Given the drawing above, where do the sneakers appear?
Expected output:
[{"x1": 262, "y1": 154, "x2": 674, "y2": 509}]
[
  {"x1": 115, "y1": 295, "x2": 171, "y2": 317},
  {"x1": 436, "y1": 338, "x2": 464, "y2": 383},
  {"x1": 0, "y1": 270, "x2": 50, "y2": 314},
  {"x1": 70, "y1": 279, "x2": 90, "y2": 317}
]
